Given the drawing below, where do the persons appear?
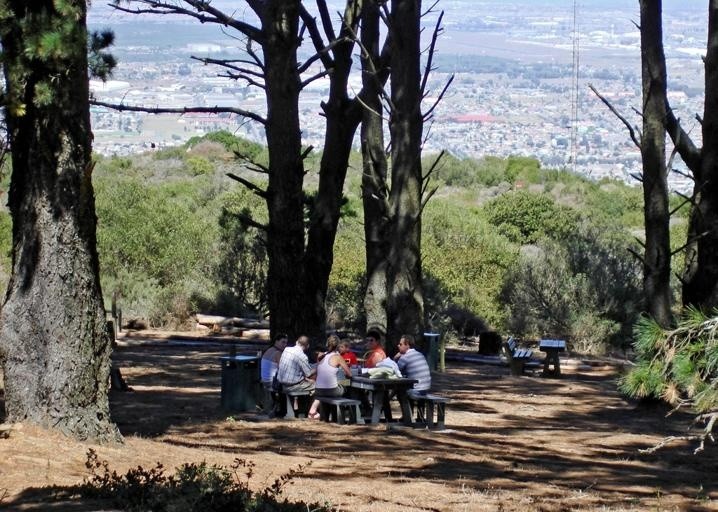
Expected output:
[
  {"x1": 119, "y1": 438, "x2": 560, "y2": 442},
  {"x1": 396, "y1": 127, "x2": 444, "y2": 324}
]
[
  {"x1": 307, "y1": 333, "x2": 353, "y2": 419},
  {"x1": 259, "y1": 333, "x2": 289, "y2": 387},
  {"x1": 393, "y1": 333, "x2": 431, "y2": 426},
  {"x1": 277, "y1": 334, "x2": 322, "y2": 420},
  {"x1": 337, "y1": 337, "x2": 357, "y2": 421},
  {"x1": 348, "y1": 330, "x2": 388, "y2": 424}
]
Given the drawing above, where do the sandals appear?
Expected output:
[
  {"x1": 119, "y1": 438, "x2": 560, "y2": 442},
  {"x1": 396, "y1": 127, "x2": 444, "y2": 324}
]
[{"x1": 308, "y1": 412, "x2": 320, "y2": 420}]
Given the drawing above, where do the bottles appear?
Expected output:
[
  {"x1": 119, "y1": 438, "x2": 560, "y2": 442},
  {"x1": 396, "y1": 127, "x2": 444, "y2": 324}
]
[{"x1": 256, "y1": 348, "x2": 261, "y2": 357}]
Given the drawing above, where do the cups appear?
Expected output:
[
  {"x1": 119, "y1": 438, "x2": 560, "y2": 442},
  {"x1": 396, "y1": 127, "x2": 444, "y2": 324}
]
[{"x1": 356, "y1": 359, "x2": 367, "y2": 376}]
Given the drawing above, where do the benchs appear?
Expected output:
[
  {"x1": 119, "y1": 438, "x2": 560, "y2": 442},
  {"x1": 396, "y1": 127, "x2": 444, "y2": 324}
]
[
  {"x1": 311, "y1": 395, "x2": 365, "y2": 425},
  {"x1": 262, "y1": 386, "x2": 311, "y2": 419},
  {"x1": 505, "y1": 336, "x2": 534, "y2": 374},
  {"x1": 405, "y1": 392, "x2": 449, "y2": 427}
]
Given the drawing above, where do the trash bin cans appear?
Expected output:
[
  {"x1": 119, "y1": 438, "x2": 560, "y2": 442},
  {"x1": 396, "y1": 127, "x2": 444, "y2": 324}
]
[
  {"x1": 220, "y1": 356, "x2": 261, "y2": 410},
  {"x1": 421, "y1": 333, "x2": 441, "y2": 371}
]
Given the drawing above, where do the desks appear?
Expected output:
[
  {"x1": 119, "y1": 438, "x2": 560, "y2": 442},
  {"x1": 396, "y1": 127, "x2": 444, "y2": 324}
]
[
  {"x1": 539, "y1": 338, "x2": 566, "y2": 376},
  {"x1": 346, "y1": 371, "x2": 418, "y2": 424}
]
